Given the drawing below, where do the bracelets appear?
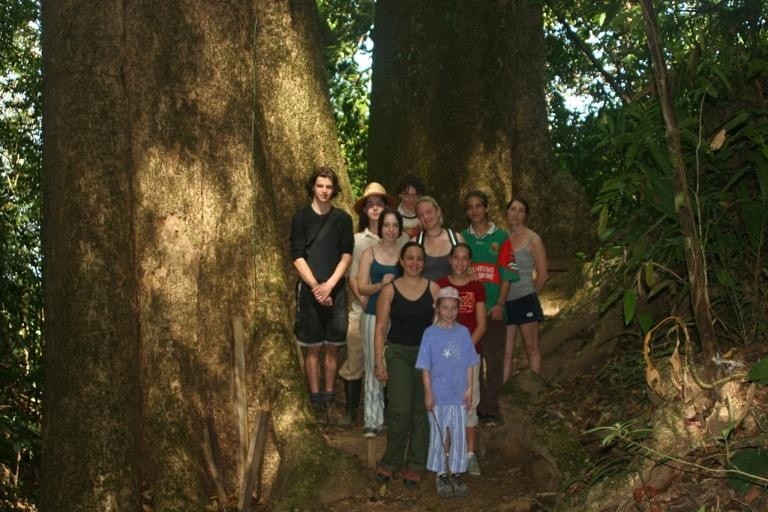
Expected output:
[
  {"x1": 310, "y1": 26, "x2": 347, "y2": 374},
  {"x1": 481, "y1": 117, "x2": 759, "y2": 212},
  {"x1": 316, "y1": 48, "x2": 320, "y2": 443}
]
[
  {"x1": 375, "y1": 365, "x2": 383, "y2": 368},
  {"x1": 495, "y1": 301, "x2": 504, "y2": 309}
]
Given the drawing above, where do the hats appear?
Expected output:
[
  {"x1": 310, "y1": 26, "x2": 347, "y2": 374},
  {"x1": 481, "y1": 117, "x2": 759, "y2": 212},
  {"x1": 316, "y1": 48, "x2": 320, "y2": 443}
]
[
  {"x1": 435, "y1": 287, "x2": 461, "y2": 304},
  {"x1": 354, "y1": 182, "x2": 397, "y2": 214}
]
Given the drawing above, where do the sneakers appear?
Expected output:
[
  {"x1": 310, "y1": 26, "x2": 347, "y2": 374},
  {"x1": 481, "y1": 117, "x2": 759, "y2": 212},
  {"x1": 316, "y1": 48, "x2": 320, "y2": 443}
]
[
  {"x1": 485, "y1": 415, "x2": 499, "y2": 427},
  {"x1": 362, "y1": 427, "x2": 377, "y2": 437},
  {"x1": 435, "y1": 452, "x2": 481, "y2": 497},
  {"x1": 374, "y1": 464, "x2": 421, "y2": 485}
]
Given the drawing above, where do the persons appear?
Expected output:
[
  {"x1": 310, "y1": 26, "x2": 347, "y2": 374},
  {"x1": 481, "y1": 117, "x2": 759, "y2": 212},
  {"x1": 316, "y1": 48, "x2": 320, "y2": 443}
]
[
  {"x1": 410, "y1": 196, "x2": 465, "y2": 282},
  {"x1": 340, "y1": 182, "x2": 396, "y2": 431},
  {"x1": 435, "y1": 243, "x2": 486, "y2": 476},
  {"x1": 396, "y1": 175, "x2": 442, "y2": 239},
  {"x1": 415, "y1": 287, "x2": 477, "y2": 499},
  {"x1": 357, "y1": 208, "x2": 402, "y2": 437},
  {"x1": 503, "y1": 196, "x2": 547, "y2": 383},
  {"x1": 373, "y1": 242, "x2": 440, "y2": 484},
  {"x1": 461, "y1": 191, "x2": 519, "y2": 428},
  {"x1": 290, "y1": 167, "x2": 354, "y2": 424}
]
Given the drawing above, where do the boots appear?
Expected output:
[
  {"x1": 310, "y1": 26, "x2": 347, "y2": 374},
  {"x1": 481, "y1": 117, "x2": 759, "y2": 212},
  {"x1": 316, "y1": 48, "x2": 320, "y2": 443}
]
[
  {"x1": 334, "y1": 378, "x2": 361, "y2": 432},
  {"x1": 310, "y1": 392, "x2": 335, "y2": 426}
]
